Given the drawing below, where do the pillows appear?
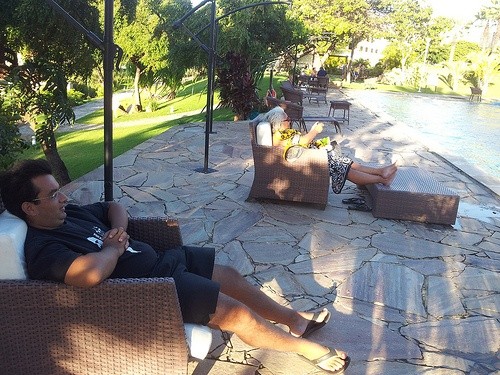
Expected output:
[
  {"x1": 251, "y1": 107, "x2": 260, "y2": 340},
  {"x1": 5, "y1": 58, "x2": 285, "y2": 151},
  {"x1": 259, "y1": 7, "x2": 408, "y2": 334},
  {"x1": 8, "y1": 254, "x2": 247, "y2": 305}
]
[
  {"x1": 257, "y1": 121, "x2": 273, "y2": 146},
  {"x1": 1, "y1": 213, "x2": 28, "y2": 280}
]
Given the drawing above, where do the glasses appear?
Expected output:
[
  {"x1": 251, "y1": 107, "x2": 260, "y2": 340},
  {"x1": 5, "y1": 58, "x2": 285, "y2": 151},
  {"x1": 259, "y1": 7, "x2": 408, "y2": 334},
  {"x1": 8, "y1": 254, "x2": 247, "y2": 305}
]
[
  {"x1": 29, "y1": 188, "x2": 63, "y2": 202},
  {"x1": 282, "y1": 118, "x2": 290, "y2": 122}
]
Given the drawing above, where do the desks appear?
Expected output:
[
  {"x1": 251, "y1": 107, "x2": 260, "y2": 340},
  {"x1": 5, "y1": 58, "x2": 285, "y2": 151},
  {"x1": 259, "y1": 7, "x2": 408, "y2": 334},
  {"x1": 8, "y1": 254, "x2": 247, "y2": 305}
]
[
  {"x1": 307, "y1": 80, "x2": 328, "y2": 106},
  {"x1": 328, "y1": 100, "x2": 352, "y2": 125}
]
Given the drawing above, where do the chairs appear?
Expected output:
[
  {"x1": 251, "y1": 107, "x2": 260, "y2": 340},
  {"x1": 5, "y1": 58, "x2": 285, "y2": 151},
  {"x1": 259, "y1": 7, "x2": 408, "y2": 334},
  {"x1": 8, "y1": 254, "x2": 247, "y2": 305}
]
[
  {"x1": 468, "y1": 86, "x2": 482, "y2": 102},
  {"x1": 279, "y1": 80, "x2": 305, "y2": 105},
  {"x1": 247, "y1": 113, "x2": 330, "y2": 206},
  {"x1": 266, "y1": 96, "x2": 309, "y2": 134},
  {"x1": 0, "y1": 217, "x2": 190, "y2": 375}
]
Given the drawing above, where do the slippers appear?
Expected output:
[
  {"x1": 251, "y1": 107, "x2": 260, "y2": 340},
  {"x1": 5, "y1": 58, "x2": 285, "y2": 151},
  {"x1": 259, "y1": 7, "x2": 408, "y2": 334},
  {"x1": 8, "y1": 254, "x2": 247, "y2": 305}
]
[
  {"x1": 342, "y1": 199, "x2": 366, "y2": 205},
  {"x1": 297, "y1": 345, "x2": 351, "y2": 374},
  {"x1": 289, "y1": 308, "x2": 331, "y2": 338},
  {"x1": 348, "y1": 204, "x2": 372, "y2": 212}
]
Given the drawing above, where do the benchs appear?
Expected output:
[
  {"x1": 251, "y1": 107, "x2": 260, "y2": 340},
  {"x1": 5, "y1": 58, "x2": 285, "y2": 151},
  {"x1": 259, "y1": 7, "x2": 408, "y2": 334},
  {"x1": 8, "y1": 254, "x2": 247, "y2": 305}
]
[{"x1": 358, "y1": 166, "x2": 459, "y2": 224}]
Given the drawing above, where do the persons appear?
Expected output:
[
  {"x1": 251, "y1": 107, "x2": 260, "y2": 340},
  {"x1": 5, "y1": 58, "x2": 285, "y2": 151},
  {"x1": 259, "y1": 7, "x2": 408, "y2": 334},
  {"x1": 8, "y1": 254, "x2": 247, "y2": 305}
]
[
  {"x1": 351, "y1": 66, "x2": 359, "y2": 81},
  {"x1": 4, "y1": 157, "x2": 352, "y2": 375},
  {"x1": 265, "y1": 106, "x2": 398, "y2": 187},
  {"x1": 303, "y1": 65, "x2": 326, "y2": 78}
]
